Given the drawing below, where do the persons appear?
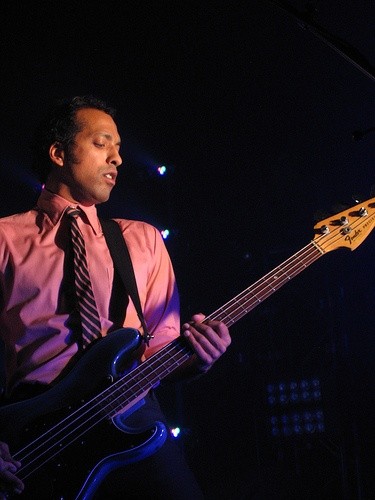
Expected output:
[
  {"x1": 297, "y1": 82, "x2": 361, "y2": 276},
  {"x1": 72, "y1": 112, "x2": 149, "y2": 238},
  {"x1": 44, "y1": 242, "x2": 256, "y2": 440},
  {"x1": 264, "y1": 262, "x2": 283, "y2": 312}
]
[{"x1": 0, "y1": 93, "x2": 231, "y2": 500}]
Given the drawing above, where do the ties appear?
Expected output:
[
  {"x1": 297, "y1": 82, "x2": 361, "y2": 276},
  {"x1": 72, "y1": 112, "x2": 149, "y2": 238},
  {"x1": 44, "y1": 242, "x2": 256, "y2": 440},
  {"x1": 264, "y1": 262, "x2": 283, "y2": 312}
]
[{"x1": 64, "y1": 206, "x2": 103, "y2": 353}]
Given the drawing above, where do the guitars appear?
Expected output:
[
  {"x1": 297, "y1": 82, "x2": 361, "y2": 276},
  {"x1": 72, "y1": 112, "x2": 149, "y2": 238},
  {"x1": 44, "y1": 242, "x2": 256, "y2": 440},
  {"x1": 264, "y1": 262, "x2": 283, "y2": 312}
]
[{"x1": 1, "y1": 196, "x2": 374, "y2": 500}]
